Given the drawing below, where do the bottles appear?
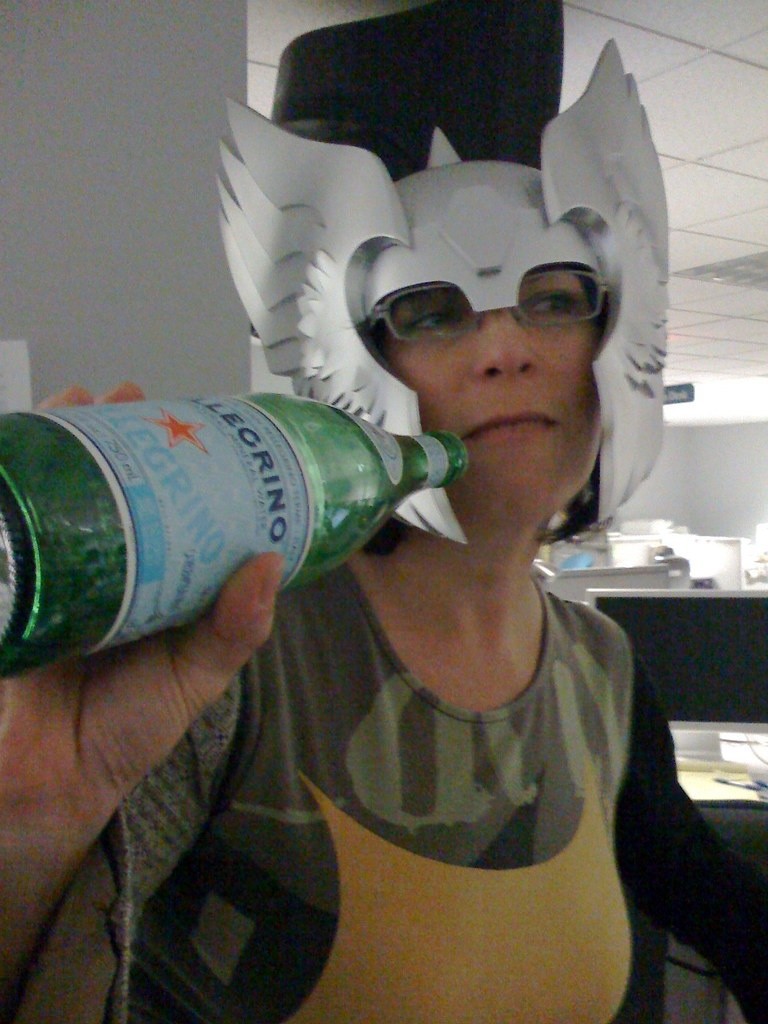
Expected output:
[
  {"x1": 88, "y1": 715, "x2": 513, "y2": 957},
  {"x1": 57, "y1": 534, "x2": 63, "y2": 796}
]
[{"x1": 0, "y1": 397, "x2": 467, "y2": 676}]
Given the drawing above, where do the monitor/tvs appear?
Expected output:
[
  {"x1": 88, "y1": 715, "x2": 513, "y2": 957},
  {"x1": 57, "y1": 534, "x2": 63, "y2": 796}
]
[{"x1": 546, "y1": 536, "x2": 768, "y2": 769}]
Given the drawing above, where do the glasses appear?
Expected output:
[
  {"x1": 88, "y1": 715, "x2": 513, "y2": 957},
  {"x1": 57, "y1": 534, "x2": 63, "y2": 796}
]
[{"x1": 370, "y1": 270, "x2": 608, "y2": 342}]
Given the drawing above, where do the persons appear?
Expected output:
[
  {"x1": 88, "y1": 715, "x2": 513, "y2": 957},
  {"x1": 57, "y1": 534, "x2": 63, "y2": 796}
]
[{"x1": 0, "y1": 37, "x2": 767, "y2": 1024}]
